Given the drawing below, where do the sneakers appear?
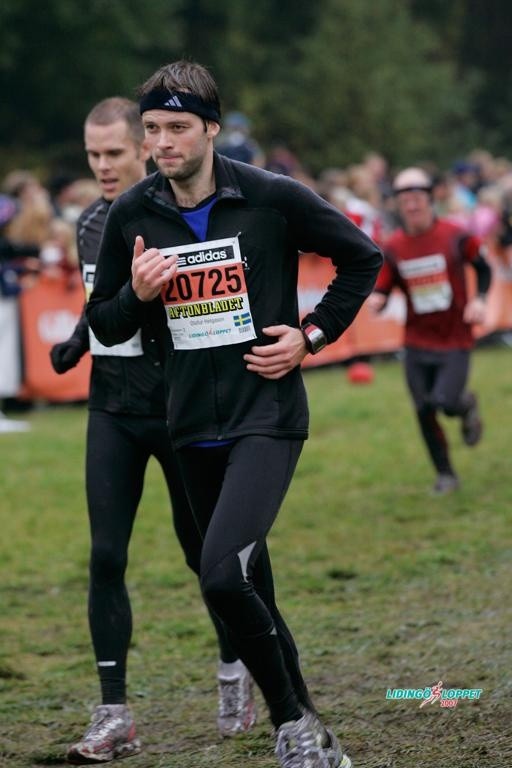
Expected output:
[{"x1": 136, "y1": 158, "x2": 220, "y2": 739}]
[
  {"x1": 461, "y1": 397, "x2": 482, "y2": 445},
  {"x1": 64, "y1": 703, "x2": 142, "y2": 765},
  {"x1": 275, "y1": 712, "x2": 352, "y2": 768},
  {"x1": 434, "y1": 473, "x2": 460, "y2": 494},
  {"x1": 217, "y1": 669, "x2": 256, "y2": 736}
]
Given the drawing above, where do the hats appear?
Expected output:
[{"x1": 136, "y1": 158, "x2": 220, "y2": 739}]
[{"x1": 392, "y1": 167, "x2": 434, "y2": 192}]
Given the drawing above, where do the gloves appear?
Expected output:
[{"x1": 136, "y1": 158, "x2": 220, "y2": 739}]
[{"x1": 49, "y1": 336, "x2": 90, "y2": 375}]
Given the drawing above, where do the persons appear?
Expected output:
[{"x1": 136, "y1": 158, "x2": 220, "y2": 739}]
[
  {"x1": 364, "y1": 165, "x2": 493, "y2": 496},
  {"x1": 0, "y1": 112, "x2": 512, "y2": 411},
  {"x1": 51, "y1": 60, "x2": 385, "y2": 767}
]
